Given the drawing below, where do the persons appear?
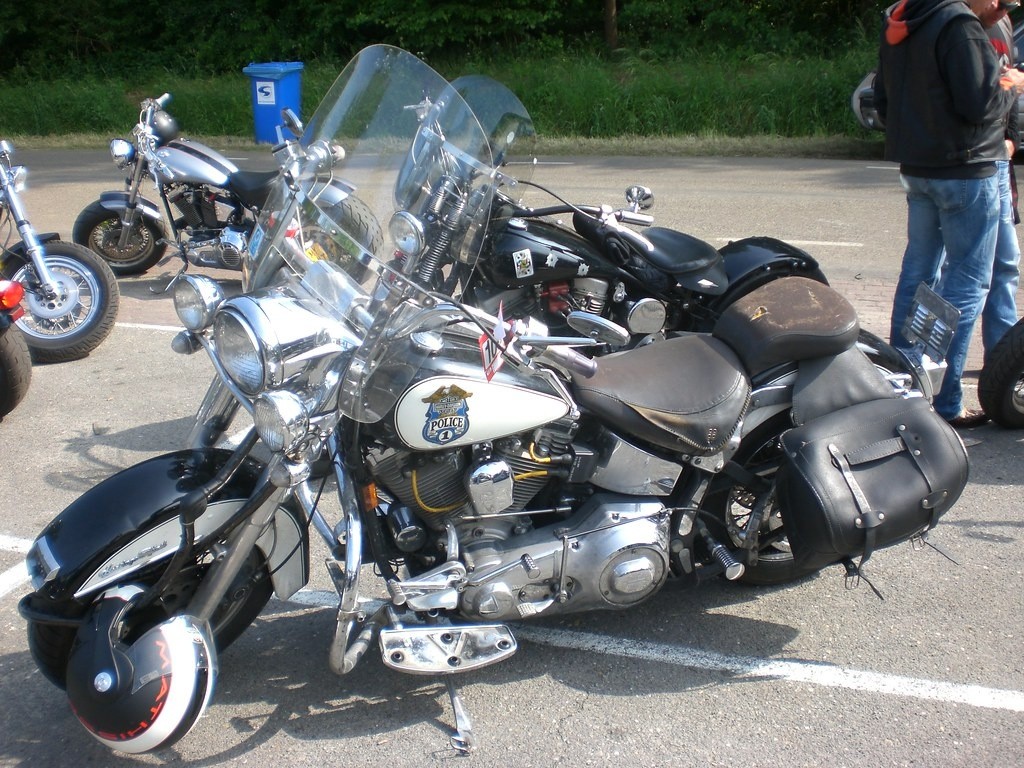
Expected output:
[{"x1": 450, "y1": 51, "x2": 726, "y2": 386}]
[{"x1": 871, "y1": 0, "x2": 1024, "y2": 429}]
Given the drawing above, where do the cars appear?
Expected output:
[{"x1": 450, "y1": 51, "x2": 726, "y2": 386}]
[{"x1": 859, "y1": 24, "x2": 1023, "y2": 151}]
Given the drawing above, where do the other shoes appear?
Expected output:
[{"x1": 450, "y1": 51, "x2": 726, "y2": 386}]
[{"x1": 950, "y1": 409, "x2": 992, "y2": 428}]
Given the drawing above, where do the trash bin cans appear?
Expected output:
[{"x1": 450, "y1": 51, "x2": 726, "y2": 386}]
[{"x1": 242, "y1": 60, "x2": 303, "y2": 144}]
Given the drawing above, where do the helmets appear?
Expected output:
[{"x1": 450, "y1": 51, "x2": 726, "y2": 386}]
[{"x1": 68, "y1": 582, "x2": 216, "y2": 755}]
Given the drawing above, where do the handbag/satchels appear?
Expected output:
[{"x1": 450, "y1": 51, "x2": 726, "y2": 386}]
[{"x1": 777, "y1": 394, "x2": 973, "y2": 574}]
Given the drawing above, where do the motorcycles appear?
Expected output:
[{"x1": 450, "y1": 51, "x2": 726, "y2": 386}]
[
  {"x1": 0, "y1": 281, "x2": 31, "y2": 416},
  {"x1": 14, "y1": 43, "x2": 969, "y2": 755},
  {"x1": 69, "y1": 91, "x2": 384, "y2": 289},
  {"x1": 341, "y1": 71, "x2": 832, "y2": 476},
  {"x1": 0, "y1": 137, "x2": 120, "y2": 367}
]
